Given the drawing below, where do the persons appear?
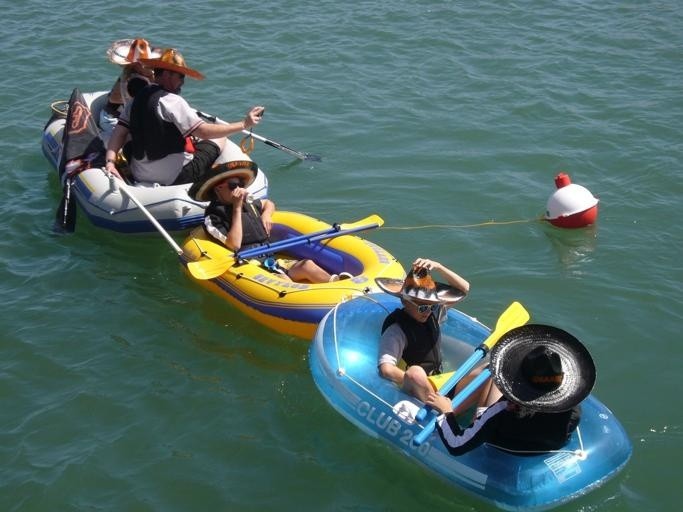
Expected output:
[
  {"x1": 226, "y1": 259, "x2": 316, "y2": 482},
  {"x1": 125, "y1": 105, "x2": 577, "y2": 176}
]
[
  {"x1": 203, "y1": 176, "x2": 354, "y2": 286},
  {"x1": 98, "y1": 62, "x2": 265, "y2": 185}
]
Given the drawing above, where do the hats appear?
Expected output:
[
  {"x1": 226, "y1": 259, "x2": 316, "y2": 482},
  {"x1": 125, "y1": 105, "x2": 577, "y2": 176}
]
[
  {"x1": 188, "y1": 160, "x2": 258, "y2": 202},
  {"x1": 374, "y1": 265, "x2": 466, "y2": 304},
  {"x1": 107, "y1": 38, "x2": 204, "y2": 80}
]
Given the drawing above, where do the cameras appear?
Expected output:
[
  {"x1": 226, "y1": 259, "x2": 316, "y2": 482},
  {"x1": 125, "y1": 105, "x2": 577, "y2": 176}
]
[{"x1": 257, "y1": 105, "x2": 265, "y2": 116}]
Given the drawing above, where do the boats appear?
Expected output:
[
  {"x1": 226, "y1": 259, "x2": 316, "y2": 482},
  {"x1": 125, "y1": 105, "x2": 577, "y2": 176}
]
[
  {"x1": 310, "y1": 293, "x2": 636, "y2": 508},
  {"x1": 38, "y1": 89, "x2": 271, "y2": 232},
  {"x1": 176, "y1": 209, "x2": 405, "y2": 343}
]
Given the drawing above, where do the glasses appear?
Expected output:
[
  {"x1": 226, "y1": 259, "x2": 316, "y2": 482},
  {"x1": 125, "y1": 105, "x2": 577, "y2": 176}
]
[
  {"x1": 408, "y1": 300, "x2": 438, "y2": 314},
  {"x1": 227, "y1": 182, "x2": 245, "y2": 191}
]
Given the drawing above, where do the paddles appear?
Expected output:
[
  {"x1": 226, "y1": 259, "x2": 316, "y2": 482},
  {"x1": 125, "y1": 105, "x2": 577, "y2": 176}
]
[
  {"x1": 237, "y1": 214, "x2": 384, "y2": 254},
  {"x1": 187, "y1": 224, "x2": 377, "y2": 280},
  {"x1": 52, "y1": 175, "x2": 77, "y2": 233},
  {"x1": 415, "y1": 300, "x2": 530, "y2": 421}
]
